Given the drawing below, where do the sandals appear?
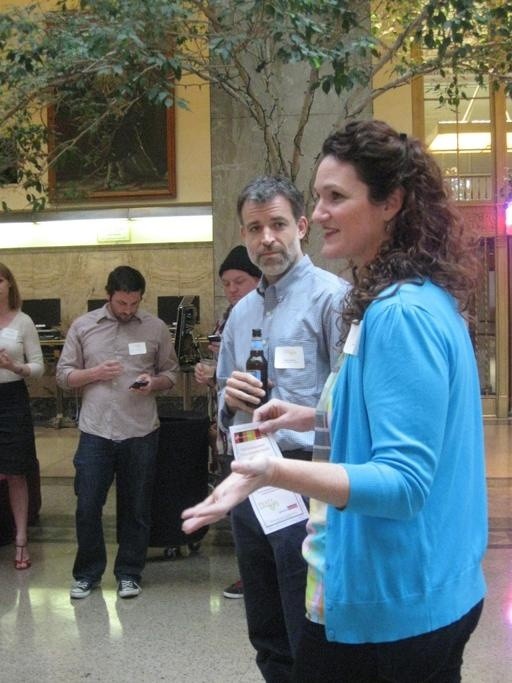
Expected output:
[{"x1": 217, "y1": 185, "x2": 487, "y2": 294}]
[{"x1": 13, "y1": 542, "x2": 31, "y2": 570}]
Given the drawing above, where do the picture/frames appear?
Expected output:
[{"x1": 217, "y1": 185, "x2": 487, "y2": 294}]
[{"x1": 40, "y1": 6, "x2": 179, "y2": 204}]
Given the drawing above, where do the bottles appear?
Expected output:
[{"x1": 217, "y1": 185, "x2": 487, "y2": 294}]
[{"x1": 246, "y1": 327, "x2": 270, "y2": 408}]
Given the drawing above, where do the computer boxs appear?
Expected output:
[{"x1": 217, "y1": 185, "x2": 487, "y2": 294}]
[{"x1": 36, "y1": 327, "x2": 61, "y2": 340}]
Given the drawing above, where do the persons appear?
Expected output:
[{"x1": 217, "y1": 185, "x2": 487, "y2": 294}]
[
  {"x1": 55, "y1": 264, "x2": 182, "y2": 599},
  {"x1": 0, "y1": 261, "x2": 47, "y2": 570},
  {"x1": 214, "y1": 172, "x2": 360, "y2": 683},
  {"x1": 193, "y1": 244, "x2": 262, "y2": 600},
  {"x1": 179, "y1": 117, "x2": 489, "y2": 682}
]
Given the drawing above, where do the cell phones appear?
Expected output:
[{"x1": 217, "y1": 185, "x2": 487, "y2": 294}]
[
  {"x1": 208, "y1": 335, "x2": 221, "y2": 342},
  {"x1": 129, "y1": 381, "x2": 149, "y2": 388}
]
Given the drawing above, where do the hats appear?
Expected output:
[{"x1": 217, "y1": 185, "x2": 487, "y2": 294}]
[{"x1": 218, "y1": 245, "x2": 263, "y2": 279}]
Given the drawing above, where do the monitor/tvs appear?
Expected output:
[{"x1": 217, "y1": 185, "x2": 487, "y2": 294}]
[
  {"x1": 21, "y1": 299, "x2": 61, "y2": 330},
  {"x1": 88, "y1": 300, "x2": 109, "y2": 313},
  {"x1": 157, "y1": 296, "x2": 200, "y2": 332}
]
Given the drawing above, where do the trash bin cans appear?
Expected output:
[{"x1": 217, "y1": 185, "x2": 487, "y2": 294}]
[{"x1": 116, "y1": 408, "x2": 211, "y2": 559}]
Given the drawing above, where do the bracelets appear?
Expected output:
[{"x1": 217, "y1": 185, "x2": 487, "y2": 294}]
[{"x1": 12, "y1": 364, "x2": 24, "y2": 376}]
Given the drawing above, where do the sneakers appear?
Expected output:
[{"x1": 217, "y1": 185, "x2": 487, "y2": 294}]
[
  {"x1": 222, "y1": 580, "x2": 245, "y2": 599},
  {"x1": 116, "y1": 578, "x2": 140, "y2": 598},
  {"x1": 70, "y1": 578, "x2": 99, "y2": 599}
]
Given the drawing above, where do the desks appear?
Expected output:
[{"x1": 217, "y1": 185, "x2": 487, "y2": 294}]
[{"x1": 37, "y1": 334, "x2": 209, "y2": 430}]
